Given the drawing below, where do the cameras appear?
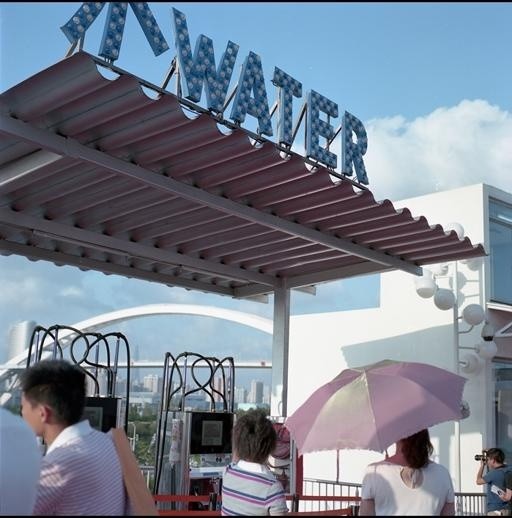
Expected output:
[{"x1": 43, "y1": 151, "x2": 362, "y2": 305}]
[{"x1": 475, "y1": 451, "x2": 488, "y2": 463}]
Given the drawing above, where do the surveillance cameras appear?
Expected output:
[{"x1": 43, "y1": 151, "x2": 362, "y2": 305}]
[{"x1": 481, "y1": 323, "x2": 495, "y2": 341}]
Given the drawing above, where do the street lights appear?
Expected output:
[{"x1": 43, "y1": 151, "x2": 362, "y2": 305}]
[{"x1": 415, "y1": 223, "x2": 497, "y2": 517}]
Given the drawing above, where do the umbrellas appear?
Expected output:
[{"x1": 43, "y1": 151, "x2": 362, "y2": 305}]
[{"x1": 282, "y1": 358, "x2": 470, "y2": 460}]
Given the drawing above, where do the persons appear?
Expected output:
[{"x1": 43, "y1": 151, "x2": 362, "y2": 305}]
[
  {"x1": 356, "y1": 427, "x2": 455, "y2": 517},
  {"x1": 18, "y1": 358, "x2": 133, "y2": 516},
  {"x1": 499, "y1": 471, "x2": 511, "y2": 505},
  {"x1": 475, "y1": 447, "x2": 511, "y2": 516},
  {"x1": 218, "y1": 410, "x2": 289, "y2": 517}
]
